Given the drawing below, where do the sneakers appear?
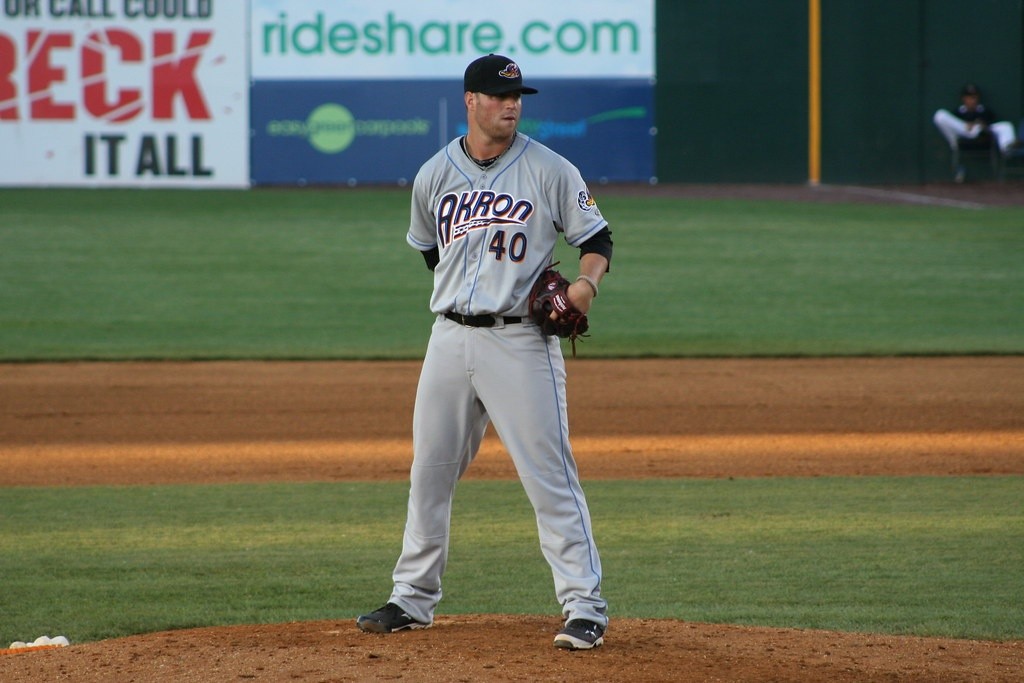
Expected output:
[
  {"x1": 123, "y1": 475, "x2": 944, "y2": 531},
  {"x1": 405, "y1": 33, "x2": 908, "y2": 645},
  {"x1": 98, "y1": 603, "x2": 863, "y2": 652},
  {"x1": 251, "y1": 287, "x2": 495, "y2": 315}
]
[
  {"x1": 553, "y1": 618, "x2": 604, "y2": 649},
  {"x1": 356, "y1": 602, "x2": 433, "y2": 633}
]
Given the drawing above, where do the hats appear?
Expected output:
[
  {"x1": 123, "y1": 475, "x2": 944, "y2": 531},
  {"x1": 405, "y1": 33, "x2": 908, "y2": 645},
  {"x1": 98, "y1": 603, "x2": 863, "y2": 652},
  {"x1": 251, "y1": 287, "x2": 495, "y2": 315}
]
[{"x1": 464, "y1": 53, "x2": 538, "y2": 95}]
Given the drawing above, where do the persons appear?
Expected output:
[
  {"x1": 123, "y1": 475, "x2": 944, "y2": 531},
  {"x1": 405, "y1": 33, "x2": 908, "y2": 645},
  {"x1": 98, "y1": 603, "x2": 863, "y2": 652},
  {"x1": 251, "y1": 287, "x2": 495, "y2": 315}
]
[
  {"x1": 956, "y1": 84, "x2": 1000, "y2": 182},
  {"x1": 357, "y1": 53, "x2": 608, "y2": 648}
]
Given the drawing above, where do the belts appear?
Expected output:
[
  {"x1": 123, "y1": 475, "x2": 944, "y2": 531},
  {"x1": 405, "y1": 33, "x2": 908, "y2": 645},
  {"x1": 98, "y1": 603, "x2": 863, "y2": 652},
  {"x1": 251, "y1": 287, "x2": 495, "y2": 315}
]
[{"x1": 445, "y1": 310, "x2": 521, "y2": 327}]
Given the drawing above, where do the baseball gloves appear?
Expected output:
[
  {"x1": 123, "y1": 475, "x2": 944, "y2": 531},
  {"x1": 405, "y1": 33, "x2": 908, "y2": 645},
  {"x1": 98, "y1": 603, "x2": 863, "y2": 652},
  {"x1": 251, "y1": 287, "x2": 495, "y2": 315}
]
[{"x1": 527, "y1": 270, "x2": 590, "y2": 339}]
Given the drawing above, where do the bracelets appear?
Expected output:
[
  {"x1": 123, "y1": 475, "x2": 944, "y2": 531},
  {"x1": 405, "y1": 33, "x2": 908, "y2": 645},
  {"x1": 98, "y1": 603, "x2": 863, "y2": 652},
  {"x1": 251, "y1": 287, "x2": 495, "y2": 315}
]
[{"x1": 576, "y1": 276, "x2": 597, "y2": 297}]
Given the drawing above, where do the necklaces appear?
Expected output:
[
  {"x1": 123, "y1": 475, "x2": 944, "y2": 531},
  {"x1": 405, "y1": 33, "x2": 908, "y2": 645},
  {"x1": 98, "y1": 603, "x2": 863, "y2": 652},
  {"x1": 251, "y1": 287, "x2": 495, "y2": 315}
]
[{"x1": 463, "y1": 130, "x2": 516, "y2": 170}]
